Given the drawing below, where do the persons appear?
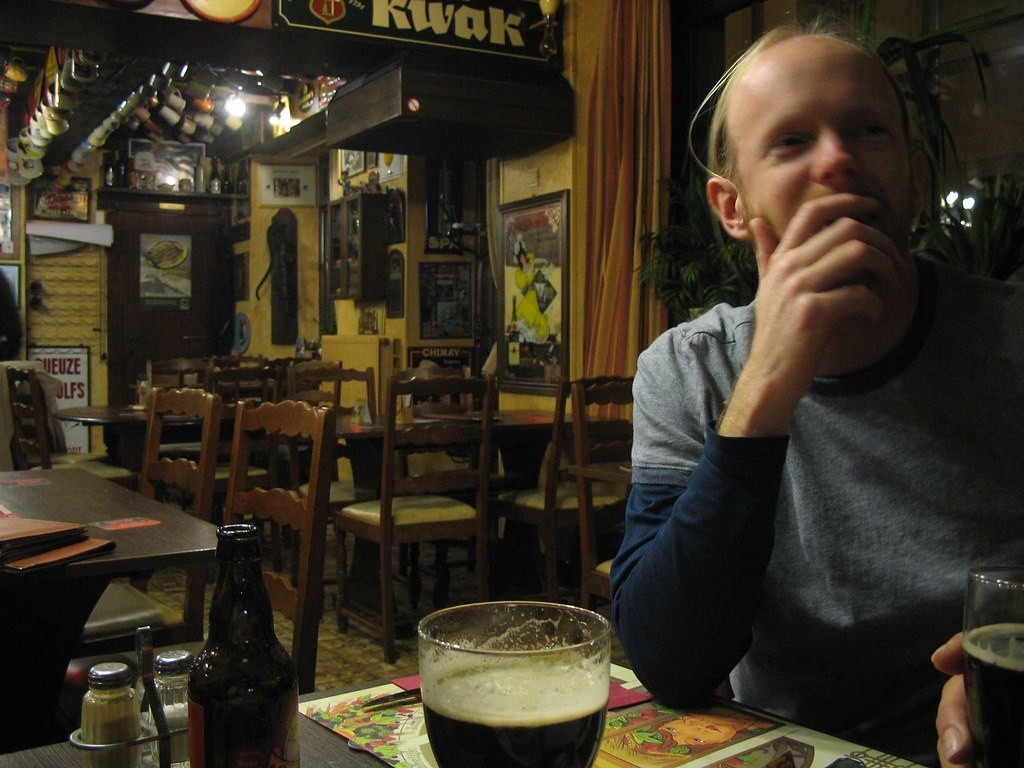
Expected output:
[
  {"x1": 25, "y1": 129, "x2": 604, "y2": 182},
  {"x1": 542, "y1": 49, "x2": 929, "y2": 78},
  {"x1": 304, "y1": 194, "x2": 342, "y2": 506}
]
[
  {"x1": 607, "y1": 24, "x2": 1024, "y2": 768},
  {"x1": 336, "y1": 169, "x2": 382, "y2": 196}
]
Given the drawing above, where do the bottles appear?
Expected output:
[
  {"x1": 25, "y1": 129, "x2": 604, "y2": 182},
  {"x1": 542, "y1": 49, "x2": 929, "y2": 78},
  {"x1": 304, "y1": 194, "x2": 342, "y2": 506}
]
[
  {"x1": 150, "y1": 650, "x2": 191, "y2": 766},
  {"x1": 187, "y1": 524, "x2": 301, "y2": 767},
  {"x1": 80, "y1": 662, "x2": 141, "y2": 768}
]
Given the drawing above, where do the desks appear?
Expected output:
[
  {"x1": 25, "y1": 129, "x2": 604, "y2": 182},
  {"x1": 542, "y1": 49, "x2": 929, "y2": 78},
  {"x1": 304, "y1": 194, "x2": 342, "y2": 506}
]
[
  {"x1": 0, "y1": 466, "x2": 225, "y2": 755},
  {"x1": 52, "y1": 402, "x2": 351, "y2": 470},
  {"x1": 333, "y1": 408, "x2": 628, "y2": 603},
  {"x1": 0, "y1": 664, "x2": 928, "y2": 768},
  {"x1": 567, "y1": 462, "x2": 633, "y2": 484}
]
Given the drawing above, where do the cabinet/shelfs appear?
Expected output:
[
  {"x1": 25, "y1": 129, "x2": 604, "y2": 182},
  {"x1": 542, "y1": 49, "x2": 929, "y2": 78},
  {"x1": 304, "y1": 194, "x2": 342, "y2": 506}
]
[{"x1": 325, "y1": 191, "x2": 386, "y2": 301}]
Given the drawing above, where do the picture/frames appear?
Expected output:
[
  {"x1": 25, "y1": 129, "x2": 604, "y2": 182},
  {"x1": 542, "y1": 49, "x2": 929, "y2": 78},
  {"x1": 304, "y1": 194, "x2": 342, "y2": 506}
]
[
  {"x1": 27, "y1": 342, "x2": 91, "y2": 455},
  {"x1": 0, "y1": 184, "x2": 21, "y2": 260},
  {"x1": 0, "y1": 262, "x2": 21, "y2": 309},
  {"x1": 418, "y1": 261, "x2": 474, "y2": 340},
  {"x1": 252, "y1": 156, "x2": 317, "y2": 208},
  {"x1": 337, "y1": 149, "x2": 365, "y2": 179},
  {"x1": 496, "y1": 189, "x2": 570, "y2": 396},
  {"x1": 128, "y1": 137, "x2": 206, "y2": 193},
  {"x1": 27, "y1": 174, "x2": 94, "y2": 224}
]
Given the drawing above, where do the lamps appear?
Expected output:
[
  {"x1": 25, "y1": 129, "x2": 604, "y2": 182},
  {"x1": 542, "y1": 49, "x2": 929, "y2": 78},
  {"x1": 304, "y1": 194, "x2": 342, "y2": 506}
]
[
  {"x1": 529, "y1": 0, "x2": 560, "y2": 57},
  {"x1": 222, "y1": 74, "x2": 246, "y2": 117}
]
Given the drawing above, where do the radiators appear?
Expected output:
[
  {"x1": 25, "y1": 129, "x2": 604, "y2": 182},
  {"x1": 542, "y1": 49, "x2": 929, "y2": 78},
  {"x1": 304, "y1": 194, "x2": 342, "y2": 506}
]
[{"x1": 321, "y1": 335, "x2": 401, "y2": 415}]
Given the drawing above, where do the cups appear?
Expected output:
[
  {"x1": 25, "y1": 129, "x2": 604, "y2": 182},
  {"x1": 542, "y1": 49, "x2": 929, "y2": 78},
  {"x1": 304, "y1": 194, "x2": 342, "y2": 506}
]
[
  {"x1": 965, "y1": 565, "x2": 1024, "y2": 768},
  {"x1": 417, "y1": 602, "x2": 611, "y2": 768}
]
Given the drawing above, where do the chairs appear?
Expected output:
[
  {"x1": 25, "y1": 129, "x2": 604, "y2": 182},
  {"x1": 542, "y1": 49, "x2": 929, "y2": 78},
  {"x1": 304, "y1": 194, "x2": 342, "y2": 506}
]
[
  {"x1": 5, "y1": 366, "x2": 133, "y2": 485},
  {"x1": 71, "y1": 385, "x2": 225, "y2": 657},
  {"x1": 289, "y1": 360, "x2": 378, "y2": 423},
  {"x1": 140, "y1": 354, "x2": 324, "y2": 421},
  {"x1": 497, "y1": 374, "x2": 632, "y2": 604},
  {"x1": 105, "y1": 397, "x2": 337, "y2": 693},
  {"x1": 337, "y1": 371, "x2": 501, "y2": 665},
  {"x1": 568, "y1": 380, "x2": 632, "y2": 609}
]
